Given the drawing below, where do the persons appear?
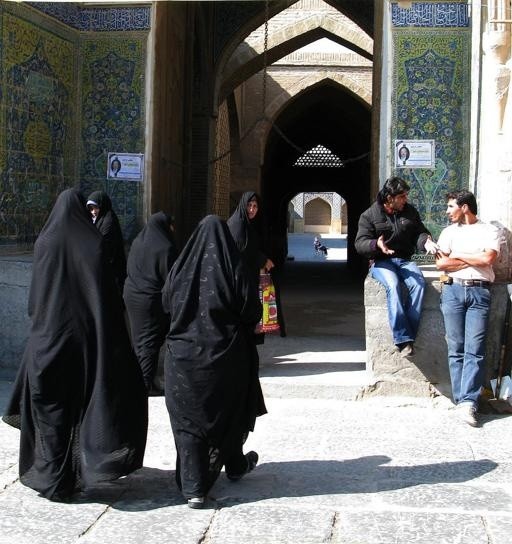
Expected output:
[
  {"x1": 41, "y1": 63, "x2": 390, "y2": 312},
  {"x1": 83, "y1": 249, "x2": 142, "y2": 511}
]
[
  {"x1": 112, "y1": 160, "x2": 121, "y2": 176},
  {"x1": 3, "y1": 186, "x2": 151, "y2": 504},
  {"x1": 157, "y1": 213, "x2": 269, "y2": 511},
  {"x1": 224, "y1": 190, "x2": 277, "y2": 345},
  {"x1": 313, "y1": 237, "x2": 321, "y2": 251},
  {"x1": 434, "y1": 189, "x2": 503, "y2": 429},
  {"x1": 354, "y1": 176, "x2": 445, "y2": 359},
  {"x1": 83, "y1": 189, "x2": 126, "y2": 272},
  {"x1": 400, "y1": 147, "x2": 409, "y2": 161},
  {"x1": 120, "y1": 210, "x2": 179, "y2": 399}
]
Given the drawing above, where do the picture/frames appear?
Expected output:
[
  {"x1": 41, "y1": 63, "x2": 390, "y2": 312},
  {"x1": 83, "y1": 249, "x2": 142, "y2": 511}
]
[
  {"x1": 394, "y1": 139, "x2": 435, "y2": 169},
  {"x1": 105, "y1": 152, "x2": 144, "y2": 182}
]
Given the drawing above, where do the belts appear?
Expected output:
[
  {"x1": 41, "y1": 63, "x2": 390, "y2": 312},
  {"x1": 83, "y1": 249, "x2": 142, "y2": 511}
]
[{"x1": 453, "y1": 278, "x2": 493, "y2": 288}]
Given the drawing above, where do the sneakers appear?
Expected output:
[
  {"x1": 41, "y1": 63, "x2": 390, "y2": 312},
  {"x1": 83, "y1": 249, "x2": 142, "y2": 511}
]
[
  {"x1": 453, "y1": 404, "x2": 479, "y2": 427},
  {"x1": 186, "y1": 498, "x2": 204, "y2": 508},
  {"x1": 392, "y1": 344, "x2": 415, "y2": 357},
  {"x1": 226, "y1": 451, "x2": 259, "y2": 481}
]
